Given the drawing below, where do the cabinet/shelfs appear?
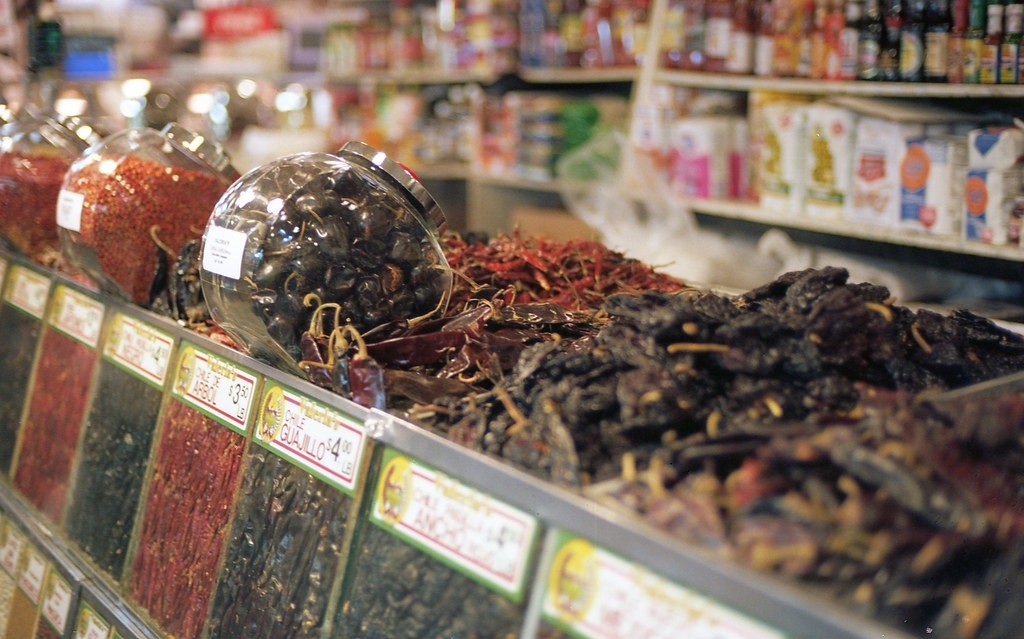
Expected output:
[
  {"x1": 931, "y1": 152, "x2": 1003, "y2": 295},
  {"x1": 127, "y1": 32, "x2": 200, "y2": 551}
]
[{"x1": 130, "y1": 0, "x2": 1024, "y2": 263}]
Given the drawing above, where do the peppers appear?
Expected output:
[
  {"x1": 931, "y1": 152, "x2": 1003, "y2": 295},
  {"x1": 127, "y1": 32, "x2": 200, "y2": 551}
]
[
  {"x1": 13, "y1": 329, "x2": 525, "y2": 639},
  {"x1": 300, "y1": 222, "x2": 1024, "y2": 638}
]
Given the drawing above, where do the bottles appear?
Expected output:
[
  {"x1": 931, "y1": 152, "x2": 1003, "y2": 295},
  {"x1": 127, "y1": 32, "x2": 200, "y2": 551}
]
[{"x1": 513, "y1": 1, "x2": 1024, "y2": 85}]
